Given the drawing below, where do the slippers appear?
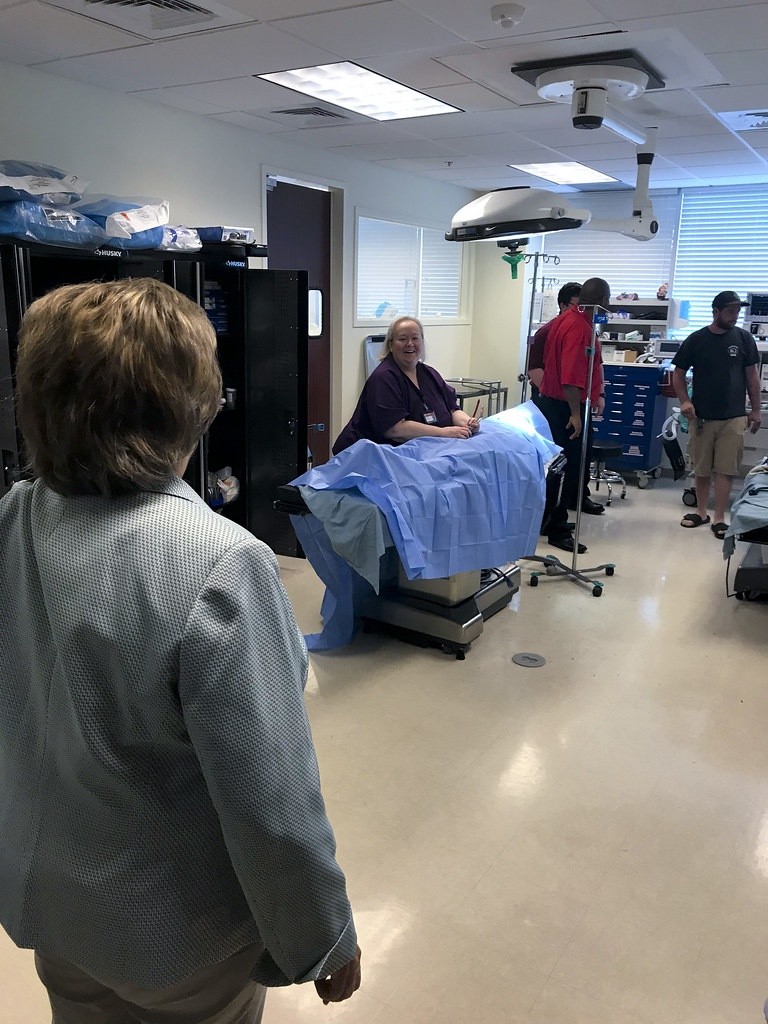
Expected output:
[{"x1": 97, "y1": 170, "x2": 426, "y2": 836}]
[
  {"x1": 680, "y1": 514, "x2": 711, "y2": 527},
  {"x1": 711, "y1": 522, "x2": 730, "y2": 539}
]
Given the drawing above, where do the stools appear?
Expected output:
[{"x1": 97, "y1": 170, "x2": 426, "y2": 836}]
[{"x1": 590, "y1": 440, "x2": 626, "y2": 506}]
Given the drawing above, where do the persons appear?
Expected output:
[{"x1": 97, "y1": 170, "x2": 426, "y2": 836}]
[
  {"x1": 331, "y1": 315, "x2": 480, "y2": 456},
  {"x1": 527, "y1": 278, "x2": 605, "y2": 552},
  {"x1": 671, "y1": 290, "x2": 761, "y2": 538},
  {"x1": 0, "y1": 279, "x2": 361, "y2": 1024}
]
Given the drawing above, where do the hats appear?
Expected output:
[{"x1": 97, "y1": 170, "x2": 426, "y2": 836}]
[{"x1": 713, "y1": 291, "x2": 750, "y2": 311}]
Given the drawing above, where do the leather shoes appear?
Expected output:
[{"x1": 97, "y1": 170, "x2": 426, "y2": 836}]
[
  {"x1": 539, "y1": 522, "x2": 575, "y2": 536},
  {"x1": 548, "y1": 535, "x2": 587, "y2": 553},
  {"x1": 567, "y1": 495, "x2": 604, "y2": 515}
]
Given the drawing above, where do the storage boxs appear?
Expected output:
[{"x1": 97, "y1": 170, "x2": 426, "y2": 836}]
[
  {"x1": 625, "y1": 330, "x2": 644, "y2": 341},
  {"x1": 650, "y1": 325, "x2": 666, "y2": 341},
  {"x1": 607, "y1": 312, "x2": 635, "y2": 319},
  {"x1": 602, "y1": 332, "x2": 618, "y2": 339},
  {"x1": 600, "y1": 344, "x2": 638, "y2": 362}
]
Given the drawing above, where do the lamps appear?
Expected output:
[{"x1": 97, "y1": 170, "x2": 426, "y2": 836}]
[{"x1": 444, "y1": 184, "x2": 659, "y2": 244}]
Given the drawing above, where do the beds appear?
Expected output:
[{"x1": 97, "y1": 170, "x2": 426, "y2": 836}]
[
  {"x1": 273, "y1": 441, "x2": 567, "y2": 662},
  {"x1": 723, "y1": 456, "x2": 768, "y2": 601}
]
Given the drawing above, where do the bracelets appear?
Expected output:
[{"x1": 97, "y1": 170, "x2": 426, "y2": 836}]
[{"x1": 600, "y1": 392, "x2": 606, "y2": 397}]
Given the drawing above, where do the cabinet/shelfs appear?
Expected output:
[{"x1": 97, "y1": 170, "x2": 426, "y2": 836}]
[
  {"x1": 0, "y1": 235, "x2": 310, "y2": 571},
  {"x1": 591, "y1": 298, "x2": 690, "y2": 489}
]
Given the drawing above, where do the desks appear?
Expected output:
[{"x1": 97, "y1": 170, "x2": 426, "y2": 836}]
[{"x1": 449, "y1": 383, "x2": 508, "y2": 416}]
